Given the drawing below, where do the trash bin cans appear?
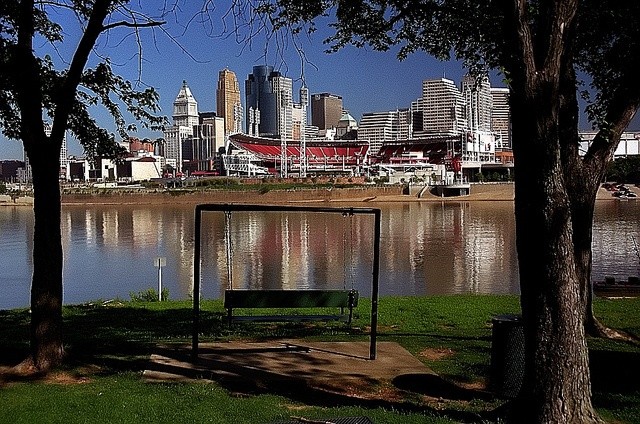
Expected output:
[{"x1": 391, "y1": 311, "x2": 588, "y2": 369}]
[{"x1": 490, "y1": 314, "x2": 526, "y2": 398}]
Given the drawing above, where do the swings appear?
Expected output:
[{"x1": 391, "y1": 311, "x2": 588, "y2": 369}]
[{"x1": 224, "y1": 209, "x2": 359, "y2": 325}]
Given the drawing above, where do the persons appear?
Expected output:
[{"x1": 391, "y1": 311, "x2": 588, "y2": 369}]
[{"x1": 399, "y1": 178, "x2": 409, "y2": 195}]
